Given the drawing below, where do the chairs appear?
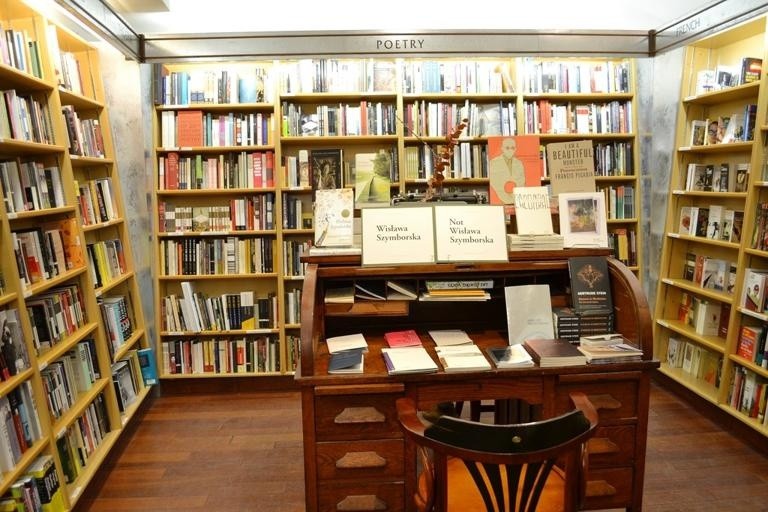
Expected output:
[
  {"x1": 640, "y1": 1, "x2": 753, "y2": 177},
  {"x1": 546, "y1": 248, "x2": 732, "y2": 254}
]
[{"x1": 393, "y1": 390, "x2": 600, "y2": 511}]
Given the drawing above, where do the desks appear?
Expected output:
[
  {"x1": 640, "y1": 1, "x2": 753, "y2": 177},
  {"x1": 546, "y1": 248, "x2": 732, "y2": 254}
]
[{"x1": 294, "y1": 247, "x2": 661, "y2": 512}]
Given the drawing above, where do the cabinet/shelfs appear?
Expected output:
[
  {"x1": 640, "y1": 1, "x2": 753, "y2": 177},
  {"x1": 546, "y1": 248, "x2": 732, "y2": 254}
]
[
  {"x1": 1, "y1": 0, "x2": 159, "y2": 512},
  {"x1": 652, "y1": 12, "x2": 768, "y2": 437},
  {"x1": 148, "y1": 56, "x2": 645, "y2": 379}
]
